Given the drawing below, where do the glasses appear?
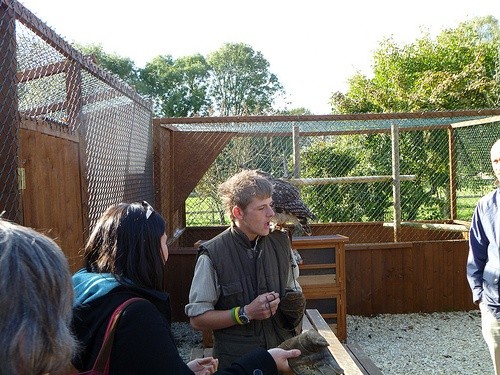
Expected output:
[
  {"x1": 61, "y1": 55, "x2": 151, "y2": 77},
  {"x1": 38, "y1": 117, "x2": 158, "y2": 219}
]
[{"x1": 141, "y1": 201, "x2": 154, "y2": 219}]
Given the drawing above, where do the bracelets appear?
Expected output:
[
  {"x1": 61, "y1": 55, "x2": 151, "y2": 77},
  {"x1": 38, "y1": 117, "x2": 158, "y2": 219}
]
[{"x1": 231, "y1": 303, "x2": 248, "y2": 326}]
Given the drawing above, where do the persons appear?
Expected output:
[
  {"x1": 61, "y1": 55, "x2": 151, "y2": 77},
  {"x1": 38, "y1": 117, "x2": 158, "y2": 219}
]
[
  {"x1": 69, "y1": 201, "x2": 301, "y2": 375},
  {"x1": 0, "y1": 211, "x2": 86, "y2": 375},
  {"x1": 184, "y1": 170, "x2": 302, "y2": 364},
  {"x1": 467, "y1": 138, "x2": 500, "y2": 375}
]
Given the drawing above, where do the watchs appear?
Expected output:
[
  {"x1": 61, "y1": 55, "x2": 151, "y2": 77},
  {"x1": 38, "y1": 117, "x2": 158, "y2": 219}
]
[{"x1": 239, "y1": 307, "x2": 251, "y2": 324}]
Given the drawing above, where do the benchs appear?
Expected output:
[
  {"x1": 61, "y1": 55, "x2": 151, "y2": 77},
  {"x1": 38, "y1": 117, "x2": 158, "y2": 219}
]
[{"x1": 342, "y1": 344, "x2": 384, "y2": 375}]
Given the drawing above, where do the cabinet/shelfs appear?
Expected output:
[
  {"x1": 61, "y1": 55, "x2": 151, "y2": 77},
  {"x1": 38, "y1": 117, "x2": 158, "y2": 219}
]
[{"x1": 195, "y1": 233, "x2": 348, "y2": 347}]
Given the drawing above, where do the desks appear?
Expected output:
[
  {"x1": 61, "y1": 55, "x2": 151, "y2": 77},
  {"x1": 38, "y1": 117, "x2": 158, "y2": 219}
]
[{"x1": 301, "y1": 309, "x2": 364, "y2": 375}]
